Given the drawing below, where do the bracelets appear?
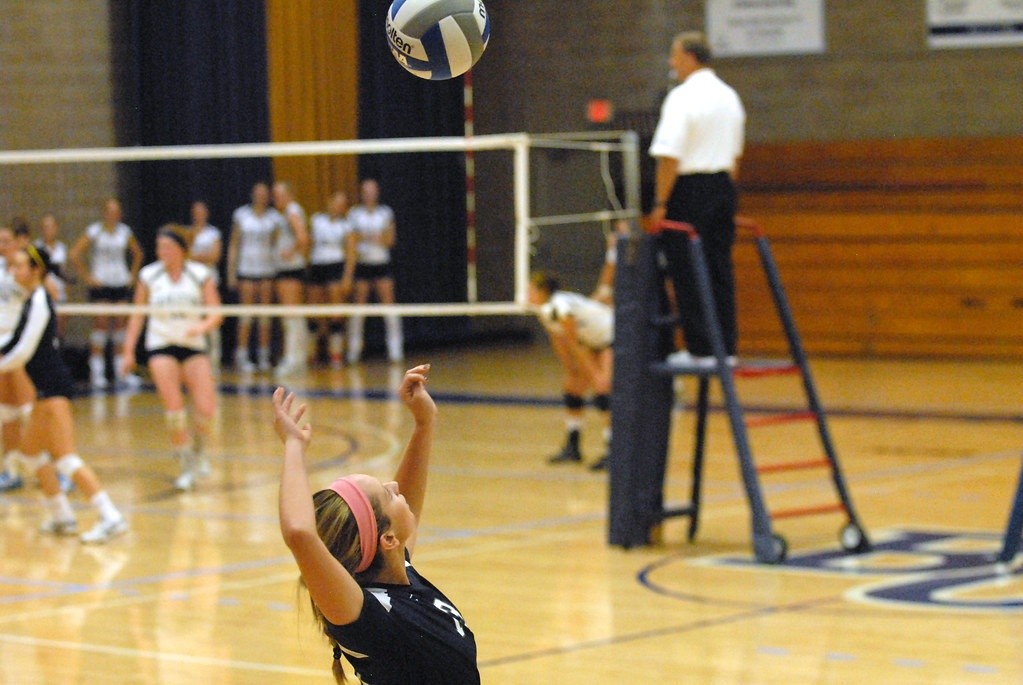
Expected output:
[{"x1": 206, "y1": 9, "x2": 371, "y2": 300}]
[{"x1": 653, "y1": 200, "x2": 667, "y2": 208}]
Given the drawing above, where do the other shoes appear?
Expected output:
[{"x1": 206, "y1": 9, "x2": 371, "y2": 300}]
[
  {"x1": 666, "y1": 349, "x2": 718, "y2": 369},
  {"x1": 304, "y1": 352, "x2": 317, "y2": 369},
  {"x1": 40, "y1": 509, "x2": 79, "y2": 535},
  {"x1": 550, "y1": 439, "x2": 582, "y2": 465},
  {"x1": 258, "y1": 357, "x2": 272, "y2": 373},
  {"x1": 0, "y1": 472, "x2": 24, "y2": 492},
  {"x1": 725, "y1": 357, "x2": 738, "y2": 371},
  {"x1": 274, "y1": 356, "x2": 305, "y2": 378},
  {"x1": 58, "y1": 474, "x2": 75, "y2": 494},
  {"x1": 347, "y1": 348, "x2": 363, "y2": 365},
  {"x1": 173, "y1": 467, "x2": 195, "y2": 491},
  {"x1": 195, "y1": 451, "x2": 210, "y2": 477},
  {"x1": 389, "y1": 340, "x2": 406, "y2": 362},
  {"x1": 90, "y1": 372, "x2": 108, "y2": 389},
  {"x1": 237, "y1": 359, "x2": 255, "y2": 375},
  {"x1": 81, "y1": 514, "x2": 128, "y2": 543},
  {"x1": 591, "y1": 440, "x2": 613, "y2": 472},
  {"x1": 115, "y1": 373, "x2": 144, "y2": 389},
  {"x1": 331, "y1": 353, "x2": 342, "y2": 372}
]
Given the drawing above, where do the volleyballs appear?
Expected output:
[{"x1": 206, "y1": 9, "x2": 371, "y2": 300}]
[{"x1": 385, "y1": 0, "x2": 492, "y2": 82}]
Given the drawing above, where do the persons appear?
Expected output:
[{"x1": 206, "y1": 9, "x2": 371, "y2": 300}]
[
  {"x1": 307, "y1": 186, "x2": 345, "y2": 365},
  {"x1": 1, "y1": 241, "x2": 129, "y2": 544},
  {"x1": 185, "y1": 202, "x2": 222, "y2": 380},
  {"x1": 69, "y1": 197, "x2": 143, "y2": 388},
  {"x1": 530, "y1": 269, "x2": 616, "y2": 471},
  {"x1": 341, "y1": 179, "x2": 404, "y2": 364},
  {"x1": 644, "y1": 31, "x2": 748, "y2": 369},
  {"x1": 0, "y1": 215, "x2": 78, "y2": 495},
  {"x1": 34, "y1": 215, "x2": 72, "y2": 344},
  {"x1": 226, "y1": 183, "x2": 288, "y2": 375},
  {"x1": 270, "y1": 363, "x2": 480, "y2": 685},
  {"x1": 119, "y1": 225, "x2": 223, "y2": 493},
  {"x1": 273, "y1": 182, "x2": 313, "y2": 375},
  {"x1": 588, "y1": 219, "x2": 630, "y2": 306}
]
